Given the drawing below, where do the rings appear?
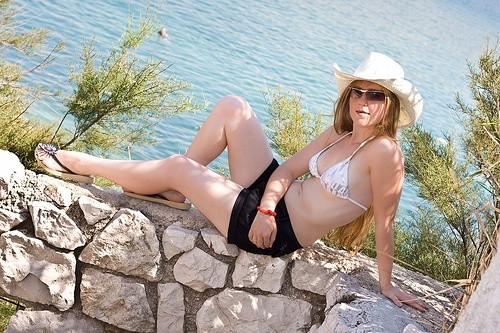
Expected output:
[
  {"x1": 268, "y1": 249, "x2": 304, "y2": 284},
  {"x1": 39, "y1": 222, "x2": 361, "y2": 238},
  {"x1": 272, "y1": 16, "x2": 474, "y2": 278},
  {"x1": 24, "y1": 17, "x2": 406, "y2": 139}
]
[{"x1": 252, "y1": 237, "x2": 257, "y2": 242}]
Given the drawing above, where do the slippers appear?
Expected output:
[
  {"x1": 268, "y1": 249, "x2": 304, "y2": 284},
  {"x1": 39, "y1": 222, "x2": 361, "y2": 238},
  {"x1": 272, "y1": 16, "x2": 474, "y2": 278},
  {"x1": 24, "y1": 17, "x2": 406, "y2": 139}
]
[
  {"x1": 35, "y1": 143, "x2": 95, "y2": 184},
  {"x1": 123, "y1": 189, "x2": 192, "y2": 211}
]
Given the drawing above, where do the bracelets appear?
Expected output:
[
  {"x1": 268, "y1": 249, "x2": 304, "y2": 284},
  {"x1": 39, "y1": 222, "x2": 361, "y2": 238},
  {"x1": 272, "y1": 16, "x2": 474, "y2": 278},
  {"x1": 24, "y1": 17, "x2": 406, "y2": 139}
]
[{"x1": 257, "y1": 206, "x2": 276, "y2": 216}]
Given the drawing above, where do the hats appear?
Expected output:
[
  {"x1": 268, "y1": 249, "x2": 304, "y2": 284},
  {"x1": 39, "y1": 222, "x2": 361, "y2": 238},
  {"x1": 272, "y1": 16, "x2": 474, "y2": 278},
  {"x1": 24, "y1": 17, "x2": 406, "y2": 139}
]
[{"x1": 332, "y1": 52, "x2": 423, "y2": 130}]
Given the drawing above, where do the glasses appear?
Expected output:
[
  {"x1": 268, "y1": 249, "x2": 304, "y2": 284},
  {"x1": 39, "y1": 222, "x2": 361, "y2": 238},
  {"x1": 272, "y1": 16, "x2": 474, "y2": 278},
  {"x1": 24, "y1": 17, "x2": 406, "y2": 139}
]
[{"x1": 349, "y1": 87, "x2": 390, "y2": 102}]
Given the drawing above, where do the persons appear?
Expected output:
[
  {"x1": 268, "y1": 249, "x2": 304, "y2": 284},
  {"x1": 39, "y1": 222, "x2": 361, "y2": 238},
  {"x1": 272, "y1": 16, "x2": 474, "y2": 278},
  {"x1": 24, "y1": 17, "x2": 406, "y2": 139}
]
[{"x1": 35, "y1": 52, "x2": 429, "y2": 313}]
